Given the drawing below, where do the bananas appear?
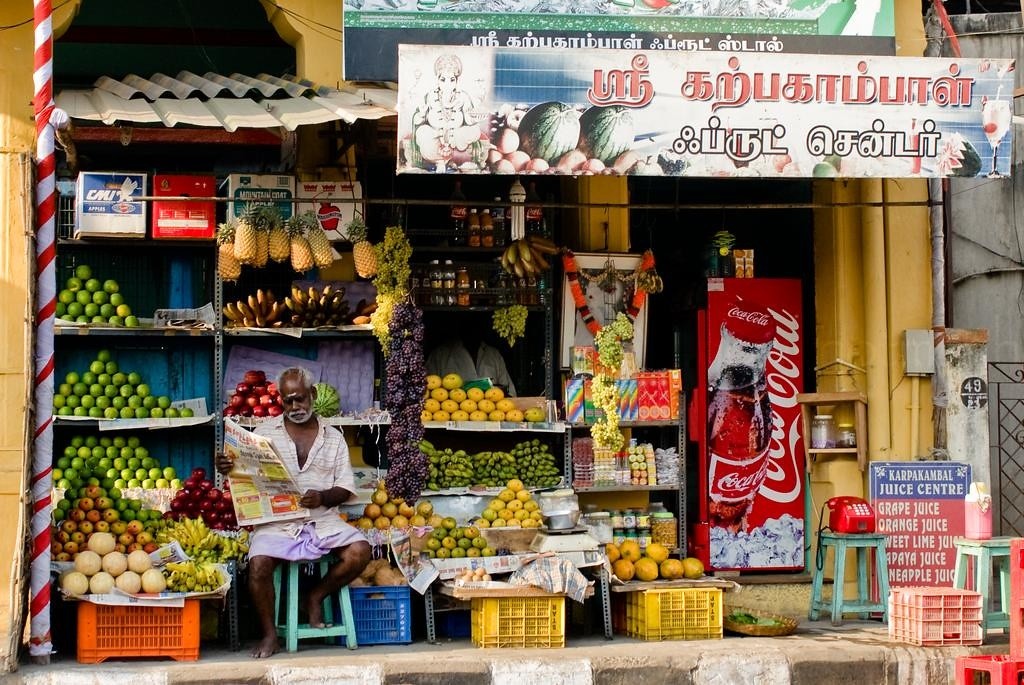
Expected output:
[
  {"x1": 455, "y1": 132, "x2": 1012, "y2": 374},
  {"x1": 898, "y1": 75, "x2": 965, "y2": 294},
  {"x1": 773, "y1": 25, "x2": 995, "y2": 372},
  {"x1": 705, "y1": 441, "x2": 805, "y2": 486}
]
[
  {"x1": 156, "y1": 515, "x2": 250, "y2": 593},
  {"x1": 223, "y1": 284, "x2": 378, "y2": 328},
  {"x1": 418, "y1": 440, "x2": 561, "y2": 492},
  {"x1": 503, "y1": 236, "x2": 559, "y2": 277}
]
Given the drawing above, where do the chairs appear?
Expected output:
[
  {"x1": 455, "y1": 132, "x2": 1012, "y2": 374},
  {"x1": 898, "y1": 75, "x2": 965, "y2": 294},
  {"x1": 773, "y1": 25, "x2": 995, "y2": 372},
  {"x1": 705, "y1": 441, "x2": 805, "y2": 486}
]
[{"x1": 273, "y1": 553, "x2": 360, "y2": 651}]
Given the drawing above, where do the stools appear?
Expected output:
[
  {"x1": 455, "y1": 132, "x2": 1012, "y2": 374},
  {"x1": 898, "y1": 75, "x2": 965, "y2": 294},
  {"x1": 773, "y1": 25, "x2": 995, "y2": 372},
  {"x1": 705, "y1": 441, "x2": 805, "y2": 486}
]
[
  {"x1": 807, "y1": 530, "x2": 889, "y2": 627},
  {"x1": 952, "y1": 534, "x2": 1017, "y2": 641}
]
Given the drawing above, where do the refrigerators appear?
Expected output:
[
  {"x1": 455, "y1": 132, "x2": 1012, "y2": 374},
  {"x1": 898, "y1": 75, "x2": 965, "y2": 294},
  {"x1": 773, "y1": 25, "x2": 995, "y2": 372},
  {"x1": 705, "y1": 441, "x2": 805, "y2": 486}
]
[{"x1": 688, "y1": 277, "x2": 805, "y2": 571}]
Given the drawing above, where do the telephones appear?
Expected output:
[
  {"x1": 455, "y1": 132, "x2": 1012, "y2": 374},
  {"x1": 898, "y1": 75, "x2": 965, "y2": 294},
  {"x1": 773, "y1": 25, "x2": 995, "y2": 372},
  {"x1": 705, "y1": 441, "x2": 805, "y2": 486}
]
[{"x1": 827, "y1": 496, "x2": 875, "y2": 534}]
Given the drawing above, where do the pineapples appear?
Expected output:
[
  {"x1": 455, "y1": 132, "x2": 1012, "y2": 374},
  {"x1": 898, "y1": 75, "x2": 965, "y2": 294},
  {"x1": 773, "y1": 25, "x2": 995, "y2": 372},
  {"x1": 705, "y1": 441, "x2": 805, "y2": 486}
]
[{"x1": 213, "y1": 202, "x2": 378, "y2": 283}]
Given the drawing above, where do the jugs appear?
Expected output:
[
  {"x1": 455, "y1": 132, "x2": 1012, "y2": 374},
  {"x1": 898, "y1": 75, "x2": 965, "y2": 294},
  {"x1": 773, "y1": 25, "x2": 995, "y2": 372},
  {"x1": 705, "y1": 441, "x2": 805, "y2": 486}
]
[{"x1": 964, "y1": 482, "x2": 992, "y2": 540}]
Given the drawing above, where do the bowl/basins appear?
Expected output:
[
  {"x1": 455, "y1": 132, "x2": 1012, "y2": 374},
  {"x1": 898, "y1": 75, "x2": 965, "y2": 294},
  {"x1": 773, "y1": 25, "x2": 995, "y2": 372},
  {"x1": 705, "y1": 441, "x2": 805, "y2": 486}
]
[{"x1": 544, "y1": 511, "x2": 581, "y2": 531}]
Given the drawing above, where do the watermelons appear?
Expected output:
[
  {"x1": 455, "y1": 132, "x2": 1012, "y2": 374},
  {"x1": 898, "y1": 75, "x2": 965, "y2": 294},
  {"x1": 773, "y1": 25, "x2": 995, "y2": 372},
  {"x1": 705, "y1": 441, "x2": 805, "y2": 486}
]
[
  {"x1": 518, "y1": 101, "x2": 580, "y2": 165},
  {"x1": 311, "y1": 383, "x2": 341, "y2": 417},
  {"x1": 579, "y1": 105, "x2": 636, "y2": 164}
]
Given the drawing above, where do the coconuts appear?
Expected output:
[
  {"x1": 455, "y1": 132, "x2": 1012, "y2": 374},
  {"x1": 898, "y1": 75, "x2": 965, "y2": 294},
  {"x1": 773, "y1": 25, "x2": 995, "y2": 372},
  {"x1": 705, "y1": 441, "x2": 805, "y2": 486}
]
[
  {"x1": 349, "y1": 559, "x2": 408, "y2": 598},
  {"x1": 606, "y1": 540, "x2": 704, "y2": 580}
]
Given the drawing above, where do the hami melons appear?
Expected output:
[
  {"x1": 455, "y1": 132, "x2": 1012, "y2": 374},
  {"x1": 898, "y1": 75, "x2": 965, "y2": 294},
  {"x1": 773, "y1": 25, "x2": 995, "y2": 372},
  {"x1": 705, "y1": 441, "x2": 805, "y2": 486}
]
[{"x1": 58, "y1": 532, "x2": 166, "y2": 595}]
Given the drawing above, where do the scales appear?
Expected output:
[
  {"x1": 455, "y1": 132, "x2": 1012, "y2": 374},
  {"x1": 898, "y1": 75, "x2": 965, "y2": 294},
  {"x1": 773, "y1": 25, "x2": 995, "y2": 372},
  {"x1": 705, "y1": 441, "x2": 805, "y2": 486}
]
[{"x1": 528, "y1": 526, "x2": 598, "y2": 553}]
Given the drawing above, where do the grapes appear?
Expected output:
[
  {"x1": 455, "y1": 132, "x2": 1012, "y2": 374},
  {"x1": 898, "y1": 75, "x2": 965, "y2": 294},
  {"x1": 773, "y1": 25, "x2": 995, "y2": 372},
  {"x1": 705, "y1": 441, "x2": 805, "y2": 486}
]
[
  {"x1": 371, "y1": 226, "x2": 429, "y2": 504},
  {"x1": 594, "y1": 312, "x2": 633, "y2": 372},
  {"x1": 590, "y1": 372, "x2": 624, "y2": 452},
  {"x1": 492, "y1": 305, "x2": 528, "y2": 346}
]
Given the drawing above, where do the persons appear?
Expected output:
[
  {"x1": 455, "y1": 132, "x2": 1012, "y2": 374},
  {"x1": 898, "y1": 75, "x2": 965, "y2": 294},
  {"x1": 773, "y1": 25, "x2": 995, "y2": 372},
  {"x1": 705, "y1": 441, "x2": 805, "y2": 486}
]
[
  {"x1": 216, "y1": 367, "x2": 372, "y2": 660},
  {"x1": 426, "y1": 320, "x2": 517, "y2": 397}
]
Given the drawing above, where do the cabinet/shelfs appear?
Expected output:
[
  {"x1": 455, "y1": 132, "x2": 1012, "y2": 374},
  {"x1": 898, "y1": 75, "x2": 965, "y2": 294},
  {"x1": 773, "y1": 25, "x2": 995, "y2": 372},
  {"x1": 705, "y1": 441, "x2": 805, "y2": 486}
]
[
  {"x1": 54, "y1": 189, "x2": 692, "y2": 646},
  {"x1": 795, "y1": 388, "x2": 867, "y2": 475}
]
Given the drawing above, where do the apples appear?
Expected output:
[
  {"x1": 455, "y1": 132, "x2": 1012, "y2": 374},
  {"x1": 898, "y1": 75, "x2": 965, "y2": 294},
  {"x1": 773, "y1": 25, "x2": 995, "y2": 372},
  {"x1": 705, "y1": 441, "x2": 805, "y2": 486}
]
[
  {"x1": 340, "y1": 480, "x2": 443, "y2": 529},
  {"x1": 50, "y1": 266, "x2": 283, "y2": 562},
  {"x1": 452, "y1": 103, "x2": 842, "y2": 177}
]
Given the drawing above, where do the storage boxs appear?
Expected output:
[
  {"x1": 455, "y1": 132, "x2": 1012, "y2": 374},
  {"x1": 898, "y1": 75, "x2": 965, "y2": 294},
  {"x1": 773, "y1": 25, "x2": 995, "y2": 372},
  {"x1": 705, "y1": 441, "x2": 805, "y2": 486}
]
[
  {"x1": 638, "y1": 371, "x2": 670, "y2": 420},
  {"x1": 218, "y1": 174, "x2": 296, "y2": 235},
  {"x1": 885, "y1": 586, "x2": 983, "y2": 647},
  {"x1": 584, "y1": 380, "x2": 595, "y2": 424},
  {"x1": 152, "y1": 174, "x2": 216, "y2": 240},
  {"x1": 621, "y1": 379, "x2": 630, "y2": 420},
  {"x1": 77, "y1": 602, "x2": 200, "y2": 663},
  {"x1": 72, "y1": 168, "x2": 149, "y2": 240},
  {"x1": 613, "y1": 378, "x2": 621, "y2": 421},
  {"x1": 470, "y1": 596, "x2": 566, "y2": 649},
  {"x1": 630, "y1": 379, "x2": 638, "y2": 421},
  {"x1": 566, "y1": 377, "x2": 584, "y2": 423},
  {"x1": 956, "y1": 654, "x2": 1024, "y2": 685},
  {"x1": 669, "y1": 369, "x2": 682, "y2": 420},
  {"x1": 625, "y1": 590, "x2": 725, "y2": 640},
  {"x1": 1009, "y1": 539, "x2": 1024, "y2": 660},
  {"x1": 295, "y1": 182, "x2": 363, "y2": 242},
  {"x1": 338, "y1": 584, "x2": 414, "y2": 646}
]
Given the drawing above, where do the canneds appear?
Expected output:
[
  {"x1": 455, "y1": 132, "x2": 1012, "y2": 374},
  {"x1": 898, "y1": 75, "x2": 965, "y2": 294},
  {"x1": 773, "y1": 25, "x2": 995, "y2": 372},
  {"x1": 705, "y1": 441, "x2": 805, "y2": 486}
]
[{"x1": 602, "y1": 507, "x2": 652, "y2": 548}]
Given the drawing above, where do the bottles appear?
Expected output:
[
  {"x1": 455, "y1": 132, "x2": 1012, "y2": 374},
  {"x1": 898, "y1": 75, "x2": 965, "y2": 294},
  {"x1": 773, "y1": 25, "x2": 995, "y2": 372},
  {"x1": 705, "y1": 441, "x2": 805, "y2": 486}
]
[
  {"x1": 812, "y1": 415, "x2": 836, "y2": 449},
  {"x1": 707, "y1": 302, "x2": 777, "y2": 532},
  {"x1": 410, "y1": 259, "x2": 539, "y2": 305},
  {"x1": 838, "y1": 424, "x2": 857, "y2": 448},
  {"x1": 450, "y1": 181, "x2": 543, "y2": 249}
]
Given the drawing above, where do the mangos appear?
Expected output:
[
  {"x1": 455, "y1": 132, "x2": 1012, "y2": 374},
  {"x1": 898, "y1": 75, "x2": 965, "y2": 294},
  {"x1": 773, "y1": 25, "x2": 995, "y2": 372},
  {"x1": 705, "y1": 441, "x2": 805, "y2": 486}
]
[
  {"x1": 423, "y1": 517, "x2": 497, "y2": 557},
  {"x1": 421, "y1": 374, "x2": 546, "y2": 421},
  {"x1": 473, "y1": 478, "x2": 543, "y2": 527}
]
